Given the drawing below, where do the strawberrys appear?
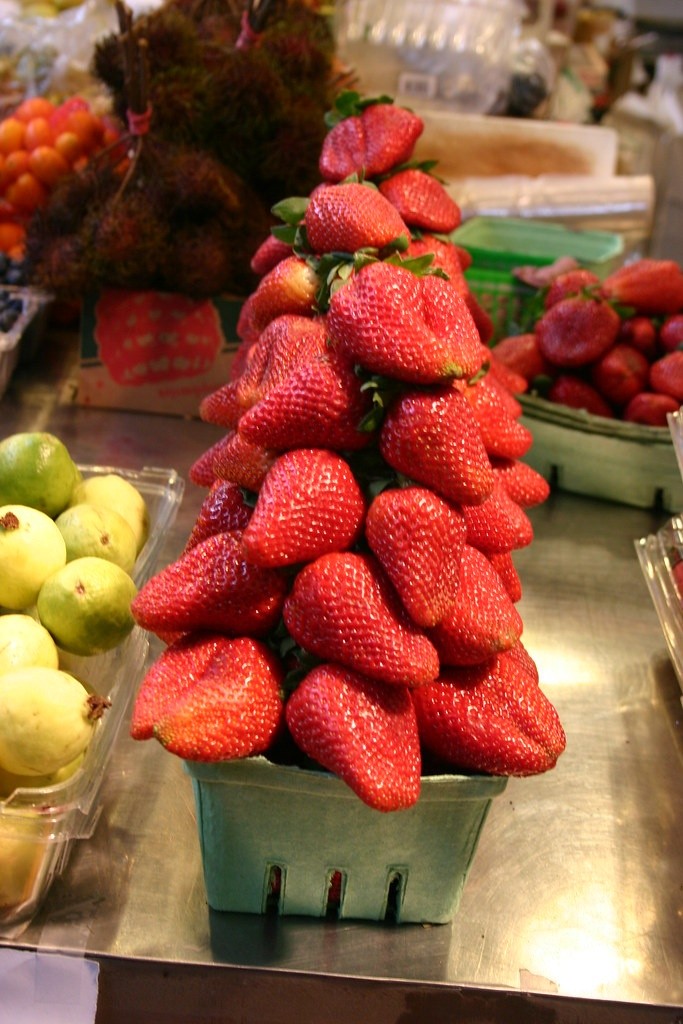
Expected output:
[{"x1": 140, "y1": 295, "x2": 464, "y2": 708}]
[{"x1": 130, "y1": 88, "x2": 683, "y2": 812}]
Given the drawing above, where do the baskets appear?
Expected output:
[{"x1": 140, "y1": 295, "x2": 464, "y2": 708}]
[
  {"x1": 514, "y1": 389, "x2": 683, "y2": 516},
  {"x1": 183, "y1": 751, "x2": 508, "y2": 925}
]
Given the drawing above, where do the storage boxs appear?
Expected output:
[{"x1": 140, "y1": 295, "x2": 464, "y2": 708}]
[{"x1": 0, "y1": 107, "x2": 683, "y2": 931}]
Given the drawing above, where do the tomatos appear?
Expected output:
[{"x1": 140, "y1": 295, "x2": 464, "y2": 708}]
[{"x1": 0, "y1": 95, "x2": 131, "y2": 266}]
